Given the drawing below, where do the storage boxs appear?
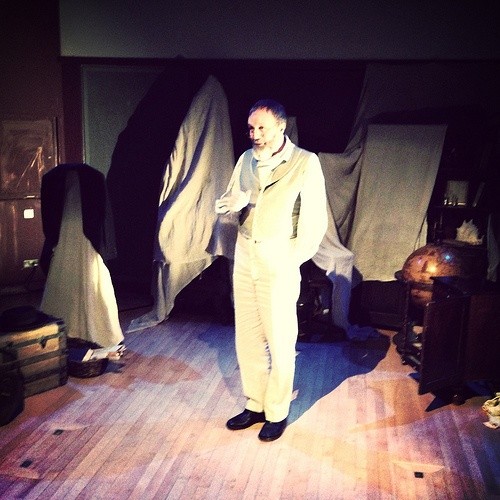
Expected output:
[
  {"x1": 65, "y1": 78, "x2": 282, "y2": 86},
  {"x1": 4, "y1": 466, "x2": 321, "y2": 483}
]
[{"x1": 1, "y1": 304, "x2": 69, "y2": 399}]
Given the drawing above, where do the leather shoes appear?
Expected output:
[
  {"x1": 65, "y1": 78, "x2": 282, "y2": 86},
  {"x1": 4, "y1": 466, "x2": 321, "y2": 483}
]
[
  {"x1": 227, "y1": 409, "x2": 266, "y2": 431},
  {"x1": 259, "y1": 417, "x2": 287, "y2": 441}
]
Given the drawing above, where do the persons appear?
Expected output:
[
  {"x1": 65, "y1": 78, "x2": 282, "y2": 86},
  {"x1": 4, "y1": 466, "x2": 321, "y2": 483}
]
[{"x1": 216, "y1": 100, "x2": 328, "y2": 440}]
